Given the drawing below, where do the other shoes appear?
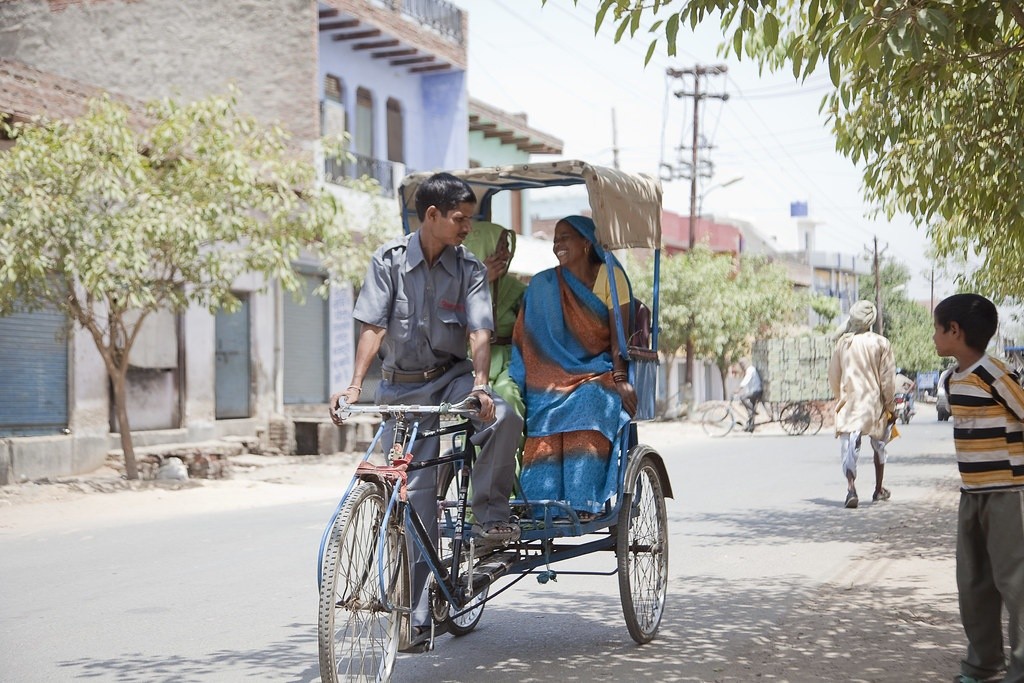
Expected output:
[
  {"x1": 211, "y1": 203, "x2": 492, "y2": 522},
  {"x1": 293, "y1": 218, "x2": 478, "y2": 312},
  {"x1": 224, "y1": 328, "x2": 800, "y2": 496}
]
[
  {"x1": 845, "y1": 493, "x2": 858, "y2": 507},
  {"x1": 873, "y1": 487, "x2": 890, "y2": 501}
]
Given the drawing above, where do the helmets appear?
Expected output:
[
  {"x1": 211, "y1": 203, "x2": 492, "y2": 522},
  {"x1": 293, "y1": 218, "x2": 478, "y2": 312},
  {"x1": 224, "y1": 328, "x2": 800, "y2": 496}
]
[{"x1": 896, "y1": 367, "x2": 908, "y2": 375}]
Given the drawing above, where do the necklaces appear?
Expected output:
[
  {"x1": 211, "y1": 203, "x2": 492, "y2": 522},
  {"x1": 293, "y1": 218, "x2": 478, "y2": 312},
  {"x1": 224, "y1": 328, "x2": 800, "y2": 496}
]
[{"x1": 585, "y1": 282, "x2": 595, "y2": 287}]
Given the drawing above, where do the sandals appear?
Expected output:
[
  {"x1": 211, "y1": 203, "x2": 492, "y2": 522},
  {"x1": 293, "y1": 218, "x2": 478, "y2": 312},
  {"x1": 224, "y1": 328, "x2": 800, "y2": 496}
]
[{"x1": 577, "y1": 513, "x2": 596, "y2": 521}]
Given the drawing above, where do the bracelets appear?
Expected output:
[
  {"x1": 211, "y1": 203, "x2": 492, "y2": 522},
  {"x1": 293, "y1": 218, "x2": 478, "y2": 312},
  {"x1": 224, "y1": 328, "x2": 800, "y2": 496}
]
[
  {"x1": 347, "y1": 385, "x2": 362, "y2": 394},
  {"x1": 612, "y1": 370, "x2": 628, "y2": 382}
]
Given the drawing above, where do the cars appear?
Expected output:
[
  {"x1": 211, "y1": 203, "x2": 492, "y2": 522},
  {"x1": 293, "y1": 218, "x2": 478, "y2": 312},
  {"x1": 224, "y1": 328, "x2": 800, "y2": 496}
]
[{"x1": 936, "y1": 387, "x2": 954, "y2": 422}]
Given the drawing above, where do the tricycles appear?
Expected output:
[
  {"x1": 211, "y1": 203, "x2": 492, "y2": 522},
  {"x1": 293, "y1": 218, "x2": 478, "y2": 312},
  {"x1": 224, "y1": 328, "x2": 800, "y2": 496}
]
[
  {"x1": 700, "y1": 386, "x2": 838, "y2": 437},
  {"x1": 316, "y1": 158, "x2": 674, "y2": 683}
]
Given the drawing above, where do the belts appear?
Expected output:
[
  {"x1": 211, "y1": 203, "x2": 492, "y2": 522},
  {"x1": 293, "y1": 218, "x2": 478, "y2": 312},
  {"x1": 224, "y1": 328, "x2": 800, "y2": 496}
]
[{"x1": 381, "y1": 366, "x2": 449, "y2": 382}]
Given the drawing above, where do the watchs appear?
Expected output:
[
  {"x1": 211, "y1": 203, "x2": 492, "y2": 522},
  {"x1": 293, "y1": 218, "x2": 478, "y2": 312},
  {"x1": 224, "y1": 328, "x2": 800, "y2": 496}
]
[{"x1": 472, "y1": 384, "x2": 493, "y2": 397}]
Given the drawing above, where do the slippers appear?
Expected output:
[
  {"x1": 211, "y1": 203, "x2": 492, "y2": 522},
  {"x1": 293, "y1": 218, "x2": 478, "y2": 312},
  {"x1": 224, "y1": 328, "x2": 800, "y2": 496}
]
[
  {"x1": 409, "y1": 624, "x2": 436, "y2": 645},
  {"x1": 471, "y1": 522, "x2": 515, "y2": 539}
]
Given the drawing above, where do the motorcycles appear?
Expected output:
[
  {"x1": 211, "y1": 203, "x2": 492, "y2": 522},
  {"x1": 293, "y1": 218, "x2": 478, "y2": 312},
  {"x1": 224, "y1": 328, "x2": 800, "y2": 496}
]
[{"x1": 895, "y1": 393, "x2": 913, "y2": 425}]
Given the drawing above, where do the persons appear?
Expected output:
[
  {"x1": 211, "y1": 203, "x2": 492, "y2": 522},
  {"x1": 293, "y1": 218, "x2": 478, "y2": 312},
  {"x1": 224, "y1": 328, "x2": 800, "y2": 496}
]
[
  {"x1": 828, "y1": 300, "x2": 896, "y2": 508},
  {"x1": 895, "y1": 367, "x2": 917, "y2": 415},
  {"x1": 459, "y1": 221, "x2": 530, "y2": 526},
  {"x1": 329, "y1": 170, "x2": 525, "y2": 654},
  {"x1": 509, "y1": 215, "x2": 638, "y2": 522},
  {"x1": 737, "y1": 355, "x2": 763, "y2": 433},
  {"x1": 933, "y1": 293, "x2": 1024, "y2": 683}
]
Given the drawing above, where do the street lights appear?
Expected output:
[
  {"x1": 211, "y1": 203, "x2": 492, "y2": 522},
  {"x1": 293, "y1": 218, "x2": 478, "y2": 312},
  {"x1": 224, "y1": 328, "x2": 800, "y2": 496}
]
[{"x1": 683, "y1": 175, "x2": 748, "y2": 383}]
